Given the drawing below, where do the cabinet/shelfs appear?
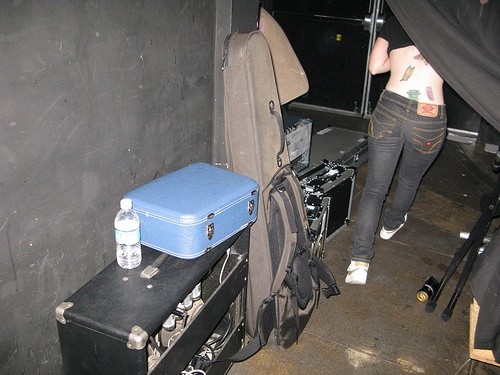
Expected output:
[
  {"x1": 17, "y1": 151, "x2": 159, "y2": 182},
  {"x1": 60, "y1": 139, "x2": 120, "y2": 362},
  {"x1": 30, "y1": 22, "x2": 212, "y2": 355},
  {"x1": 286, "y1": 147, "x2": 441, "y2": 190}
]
[{"x1": 52, "y1": 227, "x2": 249, "y2": 374}]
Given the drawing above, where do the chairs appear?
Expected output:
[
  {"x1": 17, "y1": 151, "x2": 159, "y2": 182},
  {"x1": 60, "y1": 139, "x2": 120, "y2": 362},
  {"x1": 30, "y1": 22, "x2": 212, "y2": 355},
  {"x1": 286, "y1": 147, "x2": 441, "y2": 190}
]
[{"x1": 455, "y1": 298, "x2": 500, "y2": 375}]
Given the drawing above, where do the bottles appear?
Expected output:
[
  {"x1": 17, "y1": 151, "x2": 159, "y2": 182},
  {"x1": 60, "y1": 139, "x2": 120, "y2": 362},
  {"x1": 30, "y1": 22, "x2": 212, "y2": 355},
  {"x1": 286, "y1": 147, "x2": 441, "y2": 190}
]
[{"x1": 114, "y1": 198, "x2": 141, "y2": 267}]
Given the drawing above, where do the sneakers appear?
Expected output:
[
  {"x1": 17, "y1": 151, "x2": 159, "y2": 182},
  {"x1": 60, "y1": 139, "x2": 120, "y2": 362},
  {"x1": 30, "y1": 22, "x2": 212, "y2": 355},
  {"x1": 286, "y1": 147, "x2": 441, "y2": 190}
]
[
  {"x1": 379, "y1": 213, "x2": 407, "y2": 241},
  {"x1": 345, "y1": 260, "x2": 369, "y2": 285}
]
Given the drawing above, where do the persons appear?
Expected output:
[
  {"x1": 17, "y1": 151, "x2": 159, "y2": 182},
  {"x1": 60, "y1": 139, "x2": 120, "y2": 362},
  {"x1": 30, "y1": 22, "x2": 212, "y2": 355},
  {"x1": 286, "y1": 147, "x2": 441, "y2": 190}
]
[{"x1": 345, "y1": 15, "x2": 448, "y2": 284}]
